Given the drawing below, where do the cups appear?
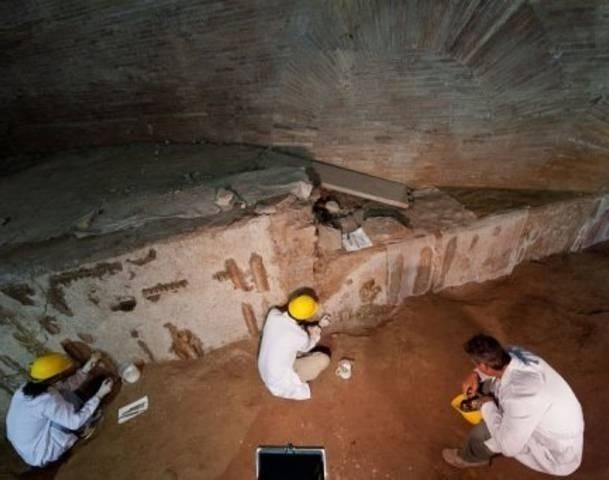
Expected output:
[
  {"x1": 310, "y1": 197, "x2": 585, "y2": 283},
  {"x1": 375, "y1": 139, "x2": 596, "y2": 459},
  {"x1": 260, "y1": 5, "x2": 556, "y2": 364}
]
[
  {"x1": 116, "y1": 360, "x2": 142, "y2": 386},
  {"x1": 336, "y1": 359, "x2": 354, "y2": 380}
]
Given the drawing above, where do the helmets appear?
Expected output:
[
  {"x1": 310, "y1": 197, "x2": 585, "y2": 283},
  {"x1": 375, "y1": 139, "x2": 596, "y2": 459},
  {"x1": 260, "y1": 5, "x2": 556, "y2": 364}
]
[
  {"x1": 28, "y1": 353, "x2": 73, "y2": 383},
  {"x1": 288, "y1": 294, "x2": 319, "y2": 320},
  {"x1": 451, "y1": 393, "x2": 482, "y2": 424}
]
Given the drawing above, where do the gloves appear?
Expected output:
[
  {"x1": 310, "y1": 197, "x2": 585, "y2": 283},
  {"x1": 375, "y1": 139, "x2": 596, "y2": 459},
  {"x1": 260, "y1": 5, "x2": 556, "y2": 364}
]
[
  {"x1": 95, "y1": 377, "x2": 114, "y2": 399},
  {"x1": 82, "y1": 351, "x2": 102, "y2": 374},
  {"x1": 318, "y1": 315, "x2": 331, "y2": 328}
]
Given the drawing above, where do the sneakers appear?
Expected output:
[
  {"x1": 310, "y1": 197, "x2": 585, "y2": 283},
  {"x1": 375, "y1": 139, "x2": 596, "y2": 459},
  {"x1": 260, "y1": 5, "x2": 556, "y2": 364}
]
[
  {"x1": 79, "y1": 409, "x2": 104, "y2": 440},
  {"x1": 442, "y1": 448, "x2": 490, "y2": 468}
]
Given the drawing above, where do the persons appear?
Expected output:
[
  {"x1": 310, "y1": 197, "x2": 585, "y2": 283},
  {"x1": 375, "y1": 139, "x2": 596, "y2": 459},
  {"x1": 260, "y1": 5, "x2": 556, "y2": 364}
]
[
  {"x1": 257, "y1": 295, "x2": 331, "y2": 400},
  {"x1": 6, "y1": 351, "x2": 115, "y2": 469},
  {"x1": 441, "y1": 334, "x2": 586, "y2": 478}
]
